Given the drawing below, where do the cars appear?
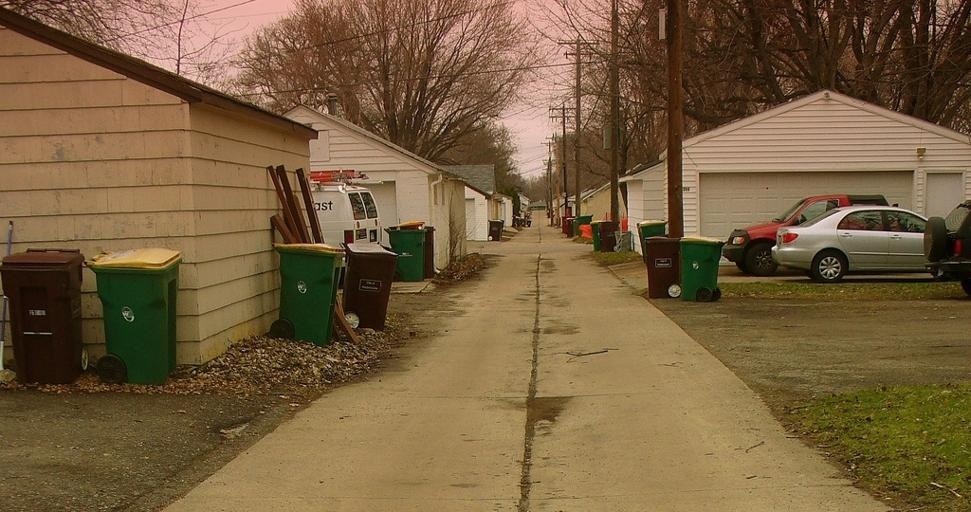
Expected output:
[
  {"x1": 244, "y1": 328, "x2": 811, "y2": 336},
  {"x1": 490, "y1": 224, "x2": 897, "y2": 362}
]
[{"x1": 771, "y1": 205, "x2": 958, "y2": 283}]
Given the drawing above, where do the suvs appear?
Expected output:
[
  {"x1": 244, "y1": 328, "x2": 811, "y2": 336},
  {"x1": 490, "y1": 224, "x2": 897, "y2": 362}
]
[
  {"x1": 923, "y1": 199, "x2": 971, "y2": 297},
  {"x1": 721, "y1": 196, "x2": 890, "y2": 277}
]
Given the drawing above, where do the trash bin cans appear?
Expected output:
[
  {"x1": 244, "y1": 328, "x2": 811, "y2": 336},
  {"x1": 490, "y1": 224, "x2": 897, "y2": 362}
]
[
  {"x1": 342, "y1": 242, "x2": 399, "y2": 331},
  {"x1": 562, "y1": 215, "x2": 593, "y2": 238},
  {"x1": 83, "y1": 247, "x2": 183, "y2": 387},
  {"x1": 489, "y1": 219, "x2": 504, "y2": 241},
  {"x1": 644, "y1": 236, "x2": 682, "y2": 298},
  {"x1": 679, "y1": 237, "x2": 727, "y2": 302},
  {"x1": 384, "y1": 221, "x2": 425, "y2": 282},
  {"x1": 598, "y1": 220, "x2": 620, "y2": 252},
  {"x1": 637, "y1": 219, "x2": 668, "y2": 263},
  {"x1": 424, "y1": 226, "x2": 435, "y2": 279},
  {"x1": 269, "y1": 242, "x2": 346, "y2": 347},
  {"x1": 590, "y1": 220, "x2": 602, "y2": 250},
  {"x1": 0, "y1": 248, "x2": 89, "y2": 386}
]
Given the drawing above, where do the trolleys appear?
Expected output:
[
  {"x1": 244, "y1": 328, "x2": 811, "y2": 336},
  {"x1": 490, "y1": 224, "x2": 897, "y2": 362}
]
[{"x1": 517, "y1": 215, "x2": 533, "y2": 228}]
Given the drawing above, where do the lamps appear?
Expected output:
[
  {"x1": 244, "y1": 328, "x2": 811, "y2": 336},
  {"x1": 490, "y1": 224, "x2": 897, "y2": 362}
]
[{"x1": 917, "y1": 147, "x2": 926, "y2": 159}]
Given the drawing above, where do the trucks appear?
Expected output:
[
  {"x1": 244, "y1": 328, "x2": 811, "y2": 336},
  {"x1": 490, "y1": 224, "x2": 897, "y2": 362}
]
[{"x1": 311, "y1": 169, "x2": 384, "y2": 289}]
